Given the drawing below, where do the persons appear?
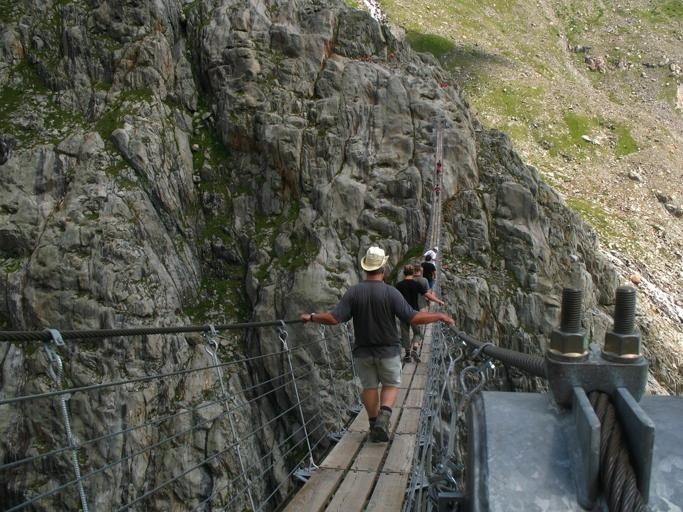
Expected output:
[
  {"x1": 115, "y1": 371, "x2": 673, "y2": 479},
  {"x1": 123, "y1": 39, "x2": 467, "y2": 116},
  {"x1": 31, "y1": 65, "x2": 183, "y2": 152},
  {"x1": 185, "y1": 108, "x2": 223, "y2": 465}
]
[
  {"x1": 393, "y1": 264, "x2": 448, "y2": 364},
  {"x1": 423, "y1": 246, "x2": 442, "y2": 269},
  {"x1": 298, "y1": 244, "x2": 456, "y2": 443},
  {"x1": 412, "y1": 261, "x2": 448, "y2": 346},
  {"x1": 419, "y1": 255, "x2": 437, "y2": 307}
]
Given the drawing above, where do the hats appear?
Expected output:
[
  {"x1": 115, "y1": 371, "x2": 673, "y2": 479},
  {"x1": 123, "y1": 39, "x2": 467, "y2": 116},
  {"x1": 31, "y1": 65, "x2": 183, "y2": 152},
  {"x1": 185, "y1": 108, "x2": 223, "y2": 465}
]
[
  {"x1": 432, "y1": 246, "x2": 438, "y2": 250},
  {"x1": 414, "y1": 264, "x2": 423, "y2": 271},
  {"x1": 361, "y1": 247, "x2": 389, "y2": 271},
  {"x1": 404, "y1": 265, "x2": 413, "y2": 270}
]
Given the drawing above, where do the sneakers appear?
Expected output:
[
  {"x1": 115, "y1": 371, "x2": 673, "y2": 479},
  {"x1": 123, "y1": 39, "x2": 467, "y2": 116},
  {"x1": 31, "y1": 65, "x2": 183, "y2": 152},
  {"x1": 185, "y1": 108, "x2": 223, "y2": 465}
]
[
  {"x1": 411, "y1": 351, "x2": 421, "y2": 363},
  {"x1": 405, "y1": 356, "x2": 411, "y2": 361}
]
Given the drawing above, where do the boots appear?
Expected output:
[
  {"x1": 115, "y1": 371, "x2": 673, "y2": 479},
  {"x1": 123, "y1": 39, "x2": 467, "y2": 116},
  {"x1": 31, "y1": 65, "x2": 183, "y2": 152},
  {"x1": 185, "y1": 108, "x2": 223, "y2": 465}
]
[
  {"x1": 374, "y1": 409, "x2": 393, "y2": 441},
  {"x1": 369, "y1": 421, "x2": 380, "y2": 443}
]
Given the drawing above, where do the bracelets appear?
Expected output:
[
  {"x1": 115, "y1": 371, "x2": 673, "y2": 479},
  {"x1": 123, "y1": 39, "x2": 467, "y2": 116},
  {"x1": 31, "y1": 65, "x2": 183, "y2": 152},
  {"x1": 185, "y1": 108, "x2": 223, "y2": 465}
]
[{"x1": 309, "y1": 311, "x2": 315, "y2": 324}]
